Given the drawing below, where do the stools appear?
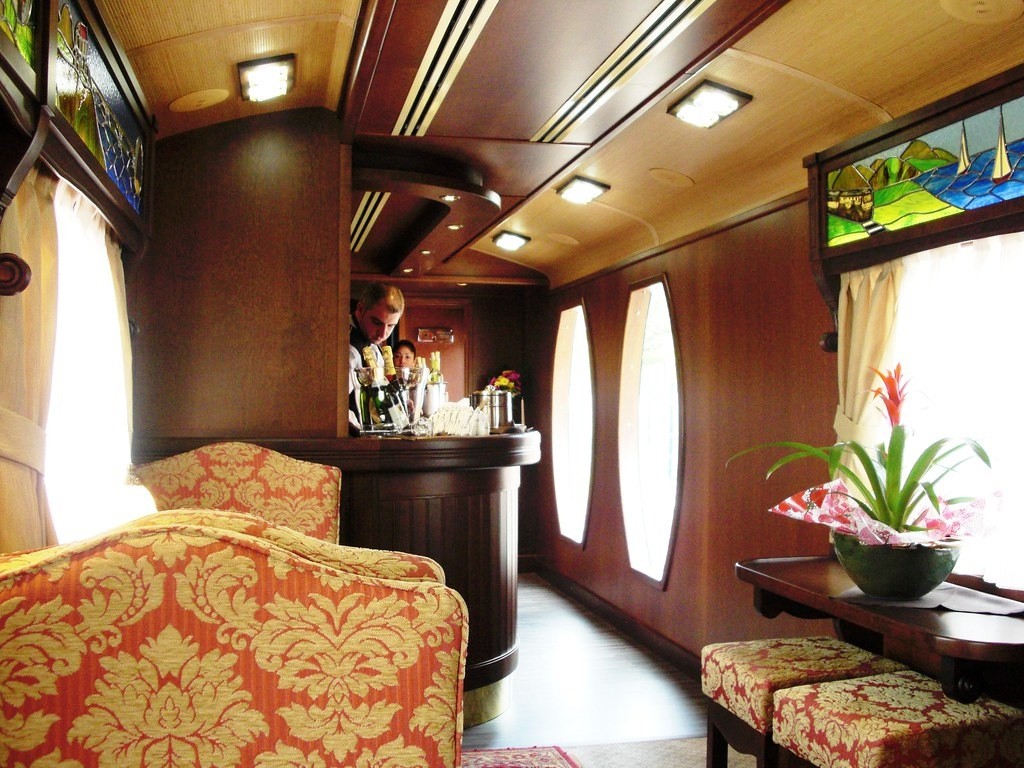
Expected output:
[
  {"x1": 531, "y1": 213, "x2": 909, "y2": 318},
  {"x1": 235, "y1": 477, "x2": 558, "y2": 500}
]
[
  {"x1": 699, "y1": 635, "x2": 911, "y2": 768},
  {"x1": 771, "y1": 671, "x2": 1024, "y2": 768}
]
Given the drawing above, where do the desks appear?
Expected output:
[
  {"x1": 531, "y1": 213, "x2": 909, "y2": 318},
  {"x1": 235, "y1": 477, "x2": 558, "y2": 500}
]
[{"x1": 735, "y1": 554, "x2": 1024, "y2": 697}]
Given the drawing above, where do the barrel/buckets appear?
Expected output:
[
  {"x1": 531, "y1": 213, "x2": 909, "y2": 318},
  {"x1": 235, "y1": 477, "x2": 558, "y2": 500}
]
[{"x1": 471, "y1": 390, "x2": 513, "y2": 433}]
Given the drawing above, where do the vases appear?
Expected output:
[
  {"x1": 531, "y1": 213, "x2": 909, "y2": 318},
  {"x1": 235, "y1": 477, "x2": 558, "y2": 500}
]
[{"x1": 830, "y1": 533, "x2": 960, "y2": 601}]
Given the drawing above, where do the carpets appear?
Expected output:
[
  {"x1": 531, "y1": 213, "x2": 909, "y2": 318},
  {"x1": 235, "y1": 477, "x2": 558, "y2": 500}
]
[{"x1": 461, "y1": 745, "x2": 585, "y2": 768}]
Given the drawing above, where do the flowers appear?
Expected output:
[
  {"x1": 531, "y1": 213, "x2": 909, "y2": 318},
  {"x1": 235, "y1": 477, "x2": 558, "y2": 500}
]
[
  {"x1": 485, "y1": 363, "x2": 523, "y2": 400},
  {"x1": 722, "y1": 360, "x2": 991, "y2": 533}
]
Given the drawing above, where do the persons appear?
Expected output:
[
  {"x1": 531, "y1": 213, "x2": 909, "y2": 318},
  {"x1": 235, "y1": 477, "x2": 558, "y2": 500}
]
[
  {"x1": 392, "y1": 339, "x2": 418, "y2": 369},
  {"x1": 347, "y1": 281, "x2": 424, "y2": 437}
]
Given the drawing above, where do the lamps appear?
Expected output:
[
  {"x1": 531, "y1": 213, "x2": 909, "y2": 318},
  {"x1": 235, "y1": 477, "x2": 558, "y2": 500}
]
[
  {"x1": 235, "y1": 53, "x2": 296, "y2": 103},
  {"x1": 492, "y1": 230, "x2": 533, "y2": 253},
  {"x1": 666, "y1": 79, "x2": 754, "y2": 129},
  {"x1": 554, "y1": 174, "x2": 612, "y2": 210}
]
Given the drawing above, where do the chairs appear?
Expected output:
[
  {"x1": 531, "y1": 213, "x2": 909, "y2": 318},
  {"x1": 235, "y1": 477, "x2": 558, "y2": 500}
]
[{"x1": 1, "y1": 442, "x2": 468, "y2": 768}]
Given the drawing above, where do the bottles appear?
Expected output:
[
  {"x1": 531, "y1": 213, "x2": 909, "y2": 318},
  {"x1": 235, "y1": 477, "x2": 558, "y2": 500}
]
[{"x1": 349, "y1": 346, "x2": 449, "y2": 435}]
